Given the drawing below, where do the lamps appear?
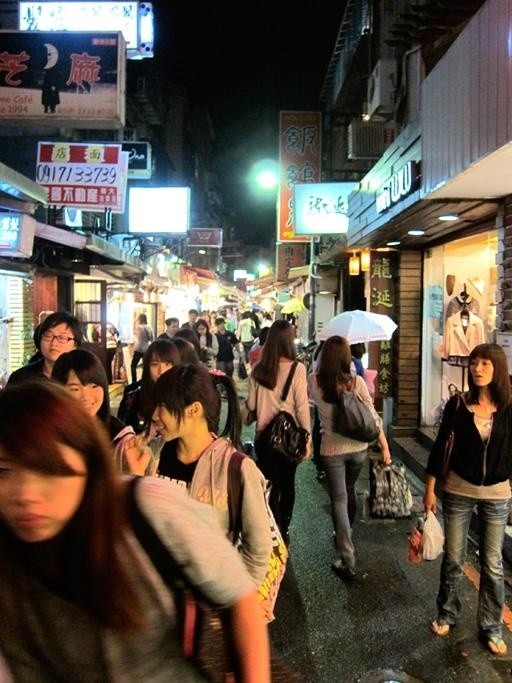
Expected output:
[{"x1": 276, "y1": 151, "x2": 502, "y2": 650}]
[
  {"x1": 359, "y1": 245, "x2": 370, "y2": 271},
  {"x1": 348, "y1": 250, "x2": 359, "y2": 275}
]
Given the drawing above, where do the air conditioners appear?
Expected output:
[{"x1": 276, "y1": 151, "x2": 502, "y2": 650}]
[
  {"x1": 347, "y1": 123, "x2": 396, "y2": 161},
  {"x1": 365, "y1": 57, "x2": 398, "y2": 123},
  {"x1": 56, "y1": 206, "x2": 83, "y2": 227}
]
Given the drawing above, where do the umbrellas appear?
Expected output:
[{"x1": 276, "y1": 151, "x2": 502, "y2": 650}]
[
  {"x1": 281, "y1": 295, "x2": 308, "y2": 316},
  {"x1": 317, "y1": 308, "x2": 399, "y2": 347}
]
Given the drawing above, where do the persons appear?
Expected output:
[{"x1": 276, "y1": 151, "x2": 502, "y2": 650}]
[
  {"x1": 2, "y1": 304, "x2": 311, "y2": 683},
  {"x1": 420, "y1": 343, "x2": 512, "y2": 659},
  {"x1": 311, "y1": 340, "x2": 362, "y2": 484},
  {"x1": 306, "y1": 334, "x2": 392, "y2": 582},
  {"x1": 352, "y1": 344, "x2": 367, "y2": 378}
]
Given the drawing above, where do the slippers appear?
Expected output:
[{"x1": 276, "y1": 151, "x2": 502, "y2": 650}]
[
  {"x1": 430, "y1": 618, "x2": 452, "y2": 638},
  {"x1": 331, "y1": 529, "x2": 354, "y2": 538},
  {"x1": 333, "y1": 559, "x2": 357, "y2": 579},
  {"x1": 478, "y1": 627, "x2": 508, "y2": 657}
]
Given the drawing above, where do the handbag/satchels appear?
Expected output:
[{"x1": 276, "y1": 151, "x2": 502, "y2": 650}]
[
  {"x1": 175, "y1": 586, "x2": 238, "y2": 683},
  {"x1": 330, "y1": 388, "x2": 381, "y2": 444},
  {"x1": 254, "y1": 409, "x2": 312, "y2": 474},
  {"x1": 434, "y1": 431, "x2": 456, "y2": 496},
  {"x1": 222, "y1": 479, "x2": 292, "y2": 627},
  {"x1": 369, "y1": 457, "x2": 414, "y2": 519}
]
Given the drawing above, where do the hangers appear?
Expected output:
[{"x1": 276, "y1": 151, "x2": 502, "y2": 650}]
[
  {"x1": 458, "y1": 291, "x2": 470, "y2": 298},
  {"x1": 459, "y1": 303, "x2": 468, "y2": 316}
]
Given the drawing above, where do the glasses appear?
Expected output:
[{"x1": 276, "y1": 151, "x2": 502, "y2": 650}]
[{"x1": 40, "y1": 331, "x2": 76, "y2": 344}]
